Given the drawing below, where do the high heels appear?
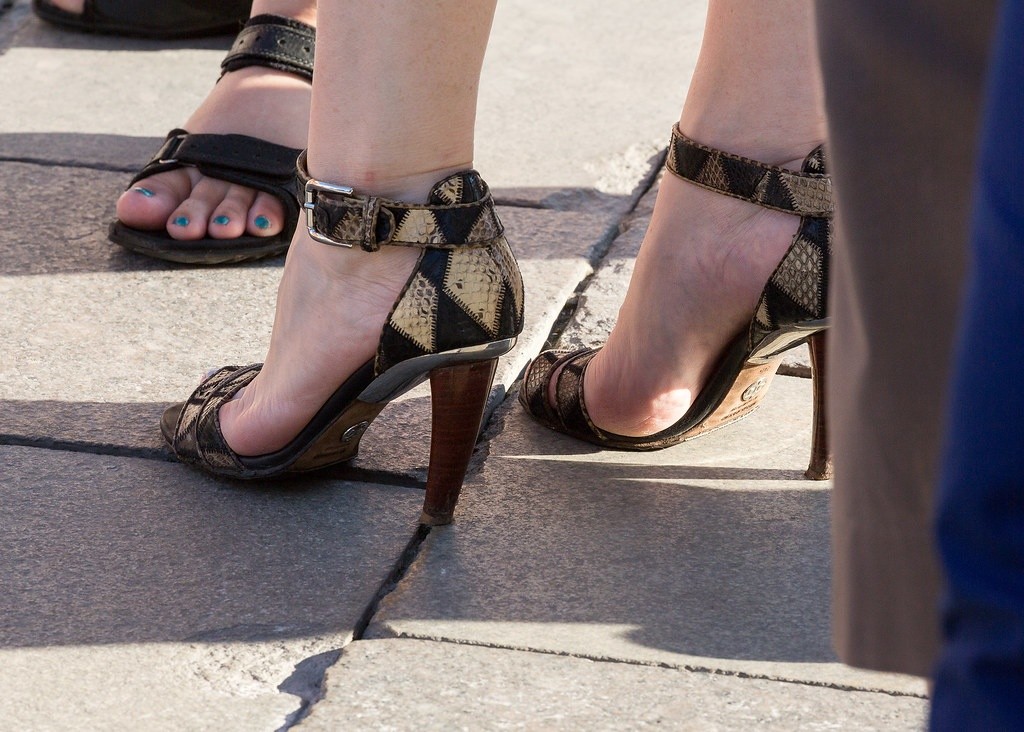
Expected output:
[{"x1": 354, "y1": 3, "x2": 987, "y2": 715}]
[
  {"x1": 160, "y1": 148, "x2": 524, "y2": 528},
  {"x1": 517, "y1": 122, "x2": 830, "y2": 480}
]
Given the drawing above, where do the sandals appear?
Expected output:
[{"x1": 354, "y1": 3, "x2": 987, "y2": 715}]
[
  {"x1": 31, "y1": 0, "x2": 251, "y2": 38},
  {"x1": 108, "y1": 14, "x2": 317, "y2": 267}
]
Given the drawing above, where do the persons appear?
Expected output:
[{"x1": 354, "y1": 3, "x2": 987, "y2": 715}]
[
  {"x1": 926, "y1": 0, "x2": 1024, "y2": 732},
  {"x1": 159, "y1": 0, "x2": 834, "y2": 527},
  {"x1": 31, "y1": 0, "x2": 318, "y2": 263}
]
